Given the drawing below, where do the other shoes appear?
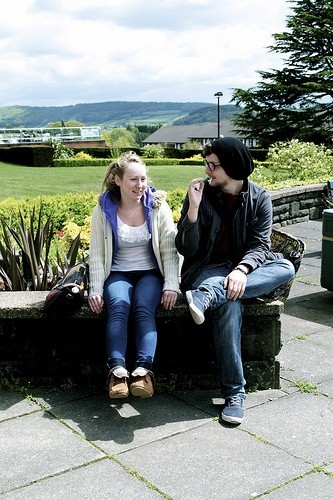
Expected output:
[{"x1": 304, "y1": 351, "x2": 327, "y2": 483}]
[
  {"x1": 110, "y1": 376, "x2": 129, "y2": 398},
  {"x1": 130, "y1": 375, "x2": 153, "y2": 398}
]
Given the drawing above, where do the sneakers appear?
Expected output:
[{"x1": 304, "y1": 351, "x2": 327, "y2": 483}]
[
  {"x1": 221, "y1": 395, "x2": 244, "y2": 425},
  {"x1": 186, "y1": 289, "x2": 213, "y2": 324}
]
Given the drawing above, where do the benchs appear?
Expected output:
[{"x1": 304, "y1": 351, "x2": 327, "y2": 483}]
[{"x1": 0, "y1": 290, "x2": 284, "y2": 388}]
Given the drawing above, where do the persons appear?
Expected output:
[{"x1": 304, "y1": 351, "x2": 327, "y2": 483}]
[
  {"x1": 86, "y1": 150, "x2": 180, "y2": 399},
  {"x1": 173, "y1": 136, "x2": 294, "y2": 425}
]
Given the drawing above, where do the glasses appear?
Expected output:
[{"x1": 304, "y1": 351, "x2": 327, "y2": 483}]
[{"x1": 203, "y1": 159, "x2": 221, "y2": 171}]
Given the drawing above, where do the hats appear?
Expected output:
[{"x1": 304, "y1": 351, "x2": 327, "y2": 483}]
[{"x1": 210, "y1": 137, "x2": 254, "y2": 181}]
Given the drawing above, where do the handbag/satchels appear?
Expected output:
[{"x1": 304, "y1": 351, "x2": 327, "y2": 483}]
[{"x1": 45, "y1": 263, "x2": 89, "y2": 309}]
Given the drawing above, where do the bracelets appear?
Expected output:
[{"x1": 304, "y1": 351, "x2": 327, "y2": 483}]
[{"x1": 234, "y1": 267, "x2": 247, "y2": 275}]
